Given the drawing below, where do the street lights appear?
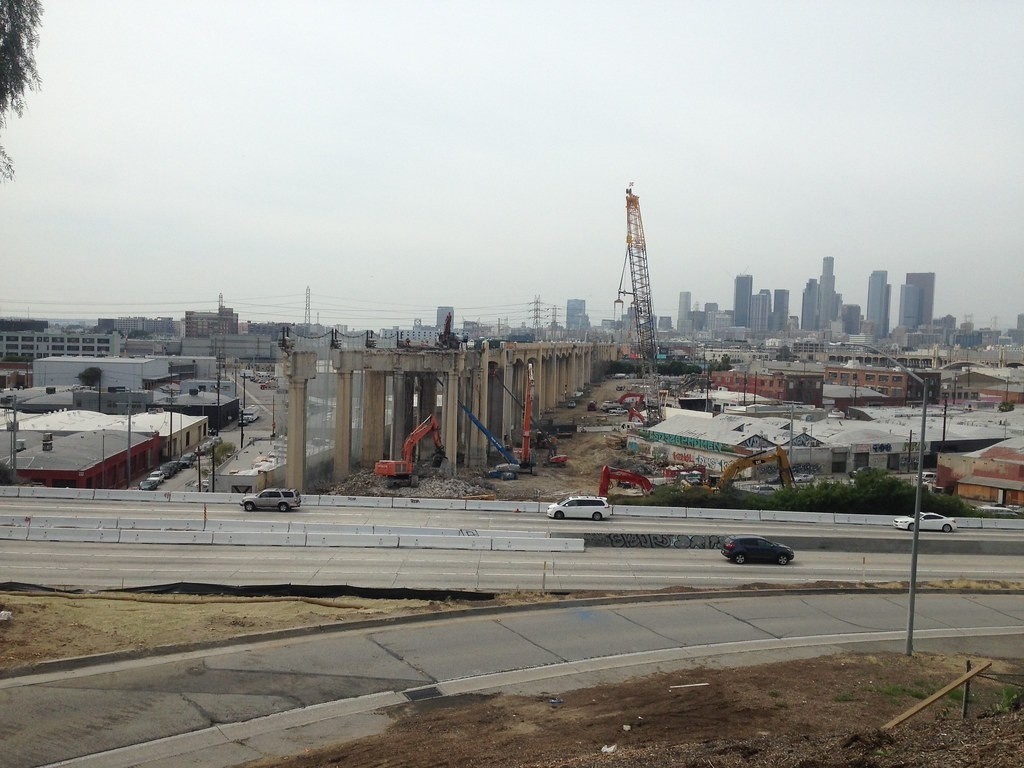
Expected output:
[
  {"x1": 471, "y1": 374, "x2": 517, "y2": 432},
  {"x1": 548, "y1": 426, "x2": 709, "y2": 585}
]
[{"x1": 826, "y1": 339, "x2": 930, "y2": 654}]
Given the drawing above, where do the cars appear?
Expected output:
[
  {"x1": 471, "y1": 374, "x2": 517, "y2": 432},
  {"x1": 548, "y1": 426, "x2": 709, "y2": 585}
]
[
  {"x1": 752, "y1": 485, "x2": 776, "y2": 494},
  {"x1": 721, "y1": 535, "x2": 795, "y2": 565},
  {"x1": 125, "y1": 367, "x2": 288, "y2": 492},
  {"x1": 792, "y1": 474, "x2": 815, "y2": 482},
  {"x1": 609, "y1": 408, "x2": 629, "y2": 414},
  {"x1": 567, "y1": 401, "x2": 577, "y2": 409},
  {"x1": 765, "y1": 474, "x2": 785, "y2": 484},
  {"x1": 588, "y1": 401, "x2": 597, "y2": 411},
  {"x1": 848, "y1": 466, "x2": 873, "y2": 478},
  {"x1": 547, "y1": 494, "x2": 611, "y2": 521},
  {"x1": 915, "y1": 472, "x2": 937, "y2": 483},
  {"x1": 892, "y1": 511, "x2": 958, "y2": 533}
]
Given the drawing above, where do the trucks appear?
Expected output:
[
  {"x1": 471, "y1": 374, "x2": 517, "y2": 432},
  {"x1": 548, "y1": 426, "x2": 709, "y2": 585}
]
[{"x1": 533, "y1": 418, "x2": 578, "y2": 439}]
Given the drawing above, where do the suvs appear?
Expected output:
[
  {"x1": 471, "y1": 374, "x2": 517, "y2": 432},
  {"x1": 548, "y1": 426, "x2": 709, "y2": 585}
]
[{"x1": 239, "y1": 488, "x2": 301, "y2": 513}]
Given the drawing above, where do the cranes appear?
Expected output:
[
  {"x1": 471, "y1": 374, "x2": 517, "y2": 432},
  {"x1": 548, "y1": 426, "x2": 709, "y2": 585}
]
[{"x1": 616, "y1": 181, "x2": 665, "y2": 430}]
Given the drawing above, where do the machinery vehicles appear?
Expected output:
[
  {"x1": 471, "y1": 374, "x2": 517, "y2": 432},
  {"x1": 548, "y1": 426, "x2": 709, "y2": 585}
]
[
  {"x1": 372, "y1": 414, "x2": 450, "y2": 491},
  {"x1": 488, "y1": 366, "x2": 570, "y2": 469},
  {"x1": 514, "y1": 363, "x2": 538, "y2": 475},
  {"x1": 599, "y1": 393, "x2": 645, "y2": 413},
  {"x1": 679, "y1": 444, "x2": 795, "y2": 496},
  {"x1": 432, "y1": 374, "x2": 522, "y2": 481},
  {"x1": 599, "y1": 464, "x2": 656, "y2": 499},
  {"x1": 620, "y1": 407, "x2": 650, "y2": 434}
]
